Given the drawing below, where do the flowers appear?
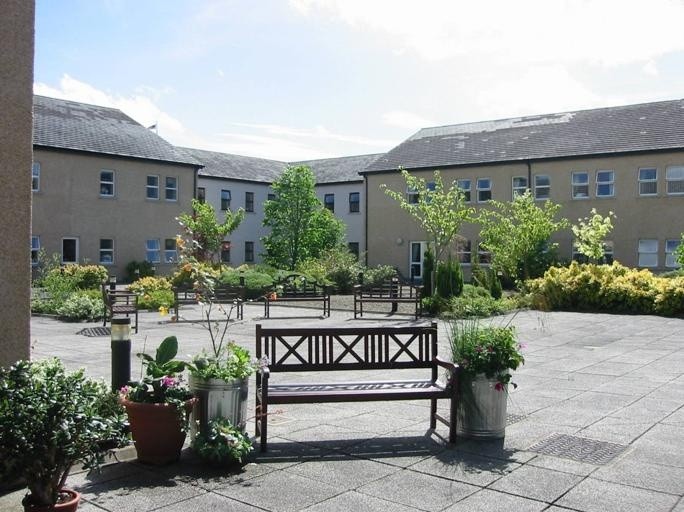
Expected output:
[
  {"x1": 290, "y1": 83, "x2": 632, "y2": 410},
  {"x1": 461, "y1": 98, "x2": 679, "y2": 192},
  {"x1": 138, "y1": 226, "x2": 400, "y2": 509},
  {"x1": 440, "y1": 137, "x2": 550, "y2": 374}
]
[
  {"x1": 191, "y1": 416, "x2": 255, "y2": 465},
  {"x1": 120, "y1": 333, "x2": 192, "y2": 403},
  {"x1": 446, "y1": 341, "x2": 529, "y2": 395},
  {"x1": 194, "y1": 341, "x2": 270, "y2": 382}
]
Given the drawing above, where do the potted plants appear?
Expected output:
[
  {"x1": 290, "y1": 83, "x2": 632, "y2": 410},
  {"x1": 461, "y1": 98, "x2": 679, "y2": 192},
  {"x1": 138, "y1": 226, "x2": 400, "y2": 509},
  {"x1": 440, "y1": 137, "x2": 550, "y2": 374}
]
[{"x1": 0, "y1": 355, "x2": 134, "y2": 512}]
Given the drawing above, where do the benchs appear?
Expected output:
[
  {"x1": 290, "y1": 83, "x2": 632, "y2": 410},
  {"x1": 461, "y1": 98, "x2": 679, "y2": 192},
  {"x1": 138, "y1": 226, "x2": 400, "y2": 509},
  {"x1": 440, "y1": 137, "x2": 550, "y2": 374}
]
[
  {"x1": 254, "y1": 321, "x2": 459, "y2": 452},
  {"x1": 263, "y1": 281, "x2": 330, "y2": 319},
  {"x1": 172, "y1": 277, "x2": 244, "y2": 320},
  {"x1": 101, "y1": 282, "x2": 138, "y2": 334},
  {"x1": 354, "y1": 284, "x2": 423, "y2": 320}
]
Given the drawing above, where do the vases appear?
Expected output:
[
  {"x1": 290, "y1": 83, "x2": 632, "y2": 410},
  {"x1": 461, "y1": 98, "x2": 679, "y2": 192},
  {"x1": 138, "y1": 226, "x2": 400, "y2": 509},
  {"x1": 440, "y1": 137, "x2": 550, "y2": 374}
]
[{"x1": 118, "y1": 395, "x2": 198, "y2": 466}]
